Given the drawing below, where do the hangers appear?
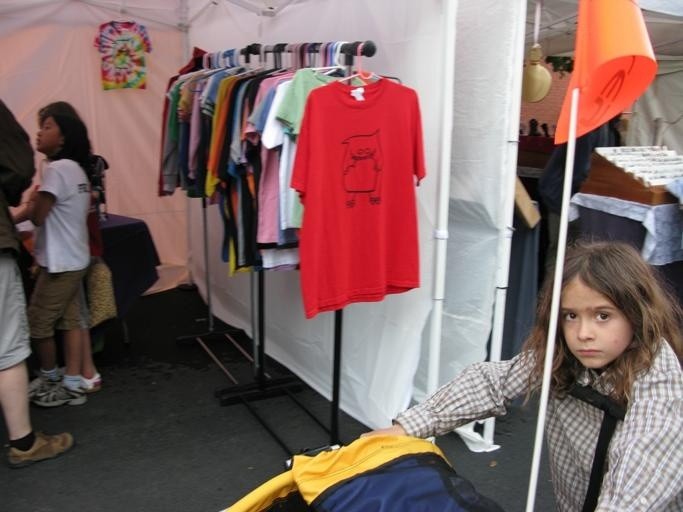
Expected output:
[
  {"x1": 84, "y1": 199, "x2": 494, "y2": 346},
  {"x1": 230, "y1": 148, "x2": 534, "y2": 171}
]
[
  {"x1": 177, "y1": 39, "x2": 402, "y2": 94},
  {"x1": 112, "y1": 6, "x2": 139, "y2": 32}
]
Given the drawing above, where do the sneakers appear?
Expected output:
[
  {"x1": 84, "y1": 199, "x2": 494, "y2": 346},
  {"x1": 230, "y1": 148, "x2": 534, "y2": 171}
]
[
  {"x1": 81, "y1": 373, "x2": 103, "y2": 394},
  {"x1": 27, "y1": 371, "x2": 64, "y2": 402},
  {"x1": 7, "y1": 428, "x2": 75, "y2": 469},
  {"x1": 31, "y1": 381, "x2": 88, "y2": 407}
]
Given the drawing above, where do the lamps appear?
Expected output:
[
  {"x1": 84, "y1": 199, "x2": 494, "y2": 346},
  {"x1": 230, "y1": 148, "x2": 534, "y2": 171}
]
[{"x1": 522, "y1": 0, "x2": 552, "y2": 103}]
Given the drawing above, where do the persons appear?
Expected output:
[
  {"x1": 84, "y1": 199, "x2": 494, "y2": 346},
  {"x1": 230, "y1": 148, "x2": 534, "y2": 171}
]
[
  {"x1": 36, "y1": 100, "x2": 103, "y2": 394},
  {"x1": 10, "y1": 100, "x2": 93, "y2": 407},
  {"x1": 0, "y1": 95, "x2": 74, "y2": 469},
  {"x1": 358, "y1": 240, "x2": 682, "y2": 512}
]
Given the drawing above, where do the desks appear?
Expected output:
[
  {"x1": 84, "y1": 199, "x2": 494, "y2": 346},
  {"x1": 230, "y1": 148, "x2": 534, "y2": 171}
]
[
  {"x1": 518, "y1": 134, "x2": 559, "y2": 169},
  {"x1": 16, "y1": 212, "x2": 148, "y2": 378}
]
